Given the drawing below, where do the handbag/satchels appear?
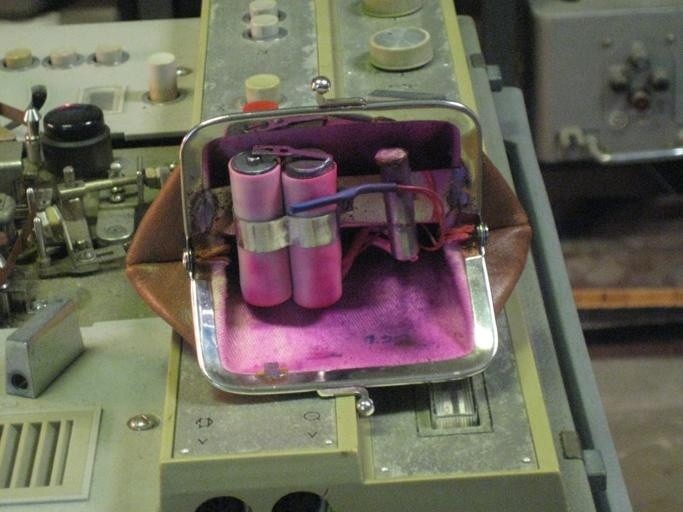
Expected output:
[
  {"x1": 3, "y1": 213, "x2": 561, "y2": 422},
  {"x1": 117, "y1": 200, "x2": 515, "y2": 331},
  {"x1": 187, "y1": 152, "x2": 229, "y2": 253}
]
[{"x1": 126, "y1": 76, "x2": 535, "y2": 418}]
